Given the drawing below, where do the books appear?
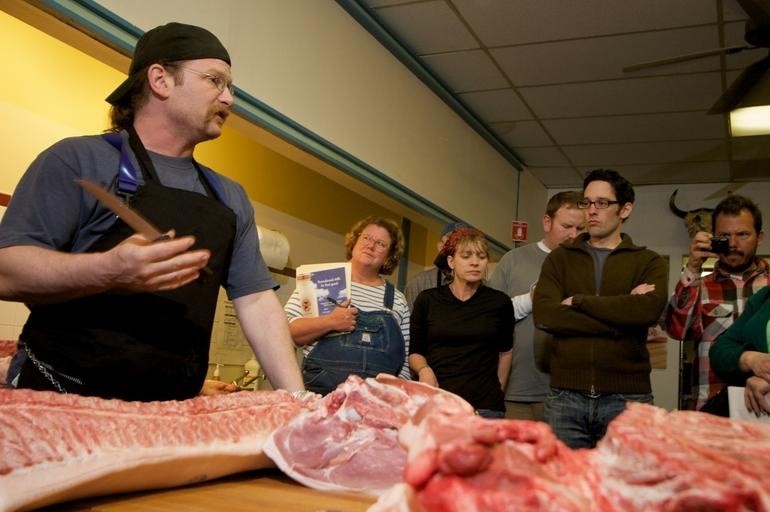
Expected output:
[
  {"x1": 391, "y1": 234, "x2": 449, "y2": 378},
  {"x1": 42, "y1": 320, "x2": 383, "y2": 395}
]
[{"x1": 292, "y1": 263, "x2": 352, "y2": 339}]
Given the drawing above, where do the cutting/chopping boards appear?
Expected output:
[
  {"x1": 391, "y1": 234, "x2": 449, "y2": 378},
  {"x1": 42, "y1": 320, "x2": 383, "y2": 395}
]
[{"x1": 25, "y1": 466, "x2": 377, "y2": 511}]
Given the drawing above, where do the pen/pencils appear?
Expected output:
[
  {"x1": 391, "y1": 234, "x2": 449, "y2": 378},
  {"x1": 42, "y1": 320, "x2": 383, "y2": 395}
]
[{"x1": 326, "y1": 297, "x2": 342, "y2": 307}]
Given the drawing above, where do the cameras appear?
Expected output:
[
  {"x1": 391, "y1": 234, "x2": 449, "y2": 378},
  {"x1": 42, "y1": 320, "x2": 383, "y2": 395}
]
[{"x1": 701, "y1": 237, "x2": 729, "y2": 254}]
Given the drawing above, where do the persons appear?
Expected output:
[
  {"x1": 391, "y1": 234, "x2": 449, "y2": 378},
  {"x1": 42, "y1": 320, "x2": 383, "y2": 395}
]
[
  {"x1": 490, "y1": 191, "x2": 587, "y2": 421},
  {"x1": 409, "y1": 228, "x2": 516, "y2": 419},
  {"x1": 0, "y1": 22, "x2": 305, "y2": 401},
  {"x1": 405, "y1": 222, "x2": 487, "y2": 312},
  {"x1": 283, "y1": 215, "x2": 412, "y2": 395},
  {"x1": 532, "y1": 168, "x2": 667, "y2": 449},
  {"x1": 663, "y1": 194, "x2": 770, "y2": 419},
  {"x1": 697, "y1": 285, "x2": 770, "y2": 418}
]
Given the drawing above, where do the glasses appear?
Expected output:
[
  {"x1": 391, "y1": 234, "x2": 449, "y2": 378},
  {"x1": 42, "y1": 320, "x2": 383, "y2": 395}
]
[
  {"x1": 356, "y1": 232, "x2": 391, "y2": 254},
  {"x1": 162, "y1": 63, "x2": 236, "y2": 96},
  {"x1": 575, "y1": 198, "x2": 620, "y2": 210}
]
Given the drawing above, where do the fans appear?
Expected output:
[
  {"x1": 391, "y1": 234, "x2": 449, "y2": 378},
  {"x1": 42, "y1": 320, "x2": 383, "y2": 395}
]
[{"x1": 623, "y1": 0, "x2": 770, "y2": 116}]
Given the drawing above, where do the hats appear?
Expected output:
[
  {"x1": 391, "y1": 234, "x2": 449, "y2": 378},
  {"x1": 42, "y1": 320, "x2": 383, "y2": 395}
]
[{"x1": 105, "y1": 22, "x2": 232, "y2": 105}]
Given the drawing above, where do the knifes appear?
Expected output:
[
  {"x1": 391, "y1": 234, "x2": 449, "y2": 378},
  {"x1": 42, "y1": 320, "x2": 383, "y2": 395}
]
[{"x1": 71, "y1": 176, "x2": 217, "y2": 279}]
